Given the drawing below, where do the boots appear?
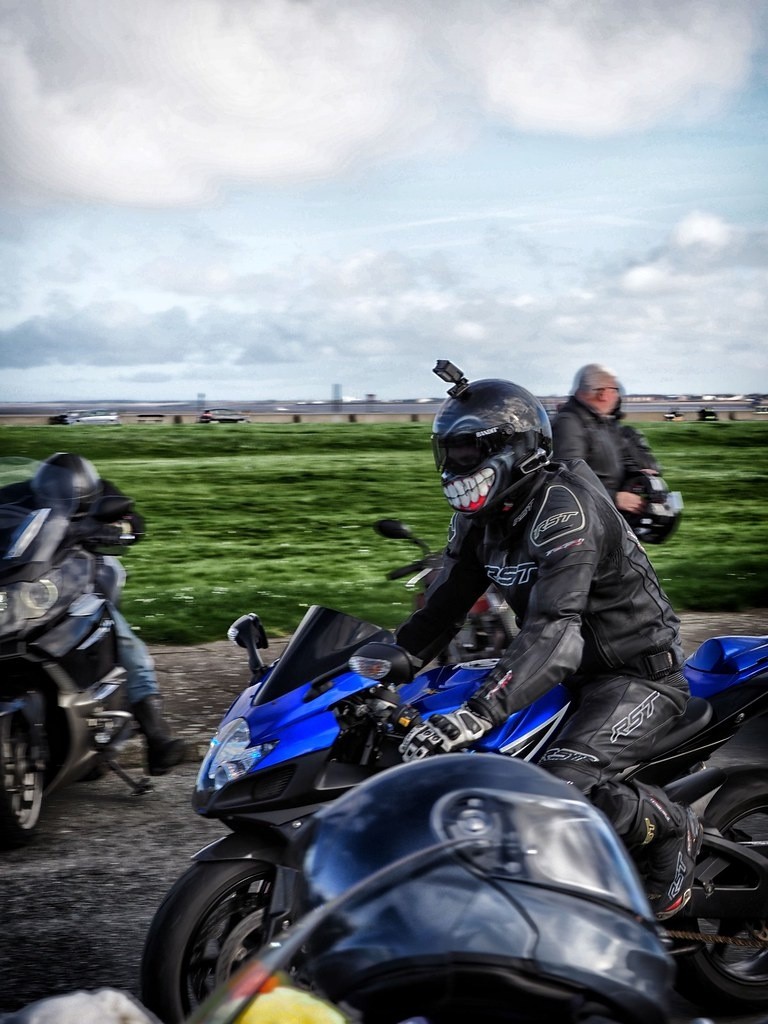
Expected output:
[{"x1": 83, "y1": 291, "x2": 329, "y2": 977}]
[
  {"x1": 621, "y1": 780, "x2": 702, "y2": 922},
  {"x1": 132, "y1": 694, "x2": 197, "y2": 775}
]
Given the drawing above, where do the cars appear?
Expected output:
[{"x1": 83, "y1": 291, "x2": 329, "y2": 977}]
[{"x1": 197, "y1": 406, "x2": 251, "y2": 425}]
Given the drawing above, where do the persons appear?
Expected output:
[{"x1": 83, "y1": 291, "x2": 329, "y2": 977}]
[
  {"x1": 547, "y1": 366, "x2": 659, "y2": 558},
  {"x1": 1, "y1": 451, "x2": 197, "y2": 780},
  {"x1": 395, "y1": 379, "x2": 701, "y2": 918}
]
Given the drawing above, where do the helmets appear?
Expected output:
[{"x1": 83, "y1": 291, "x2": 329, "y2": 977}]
[
  {"x1": 432, "y1": 380, "x2": 553, "y2": 520},
  {"x1": 279, "y1": 753, "x2": 673, "y2": 1024},
  {"x1": 31, "y1": 451, "x2": 103, "y2": 518},
  {"x1": 621, "y1": 473, "x2": 682, "y2": 545}
]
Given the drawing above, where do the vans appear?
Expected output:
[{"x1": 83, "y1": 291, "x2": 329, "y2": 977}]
[{"x1": 47, "y1": 409, "x2": 121, "y2": 427}]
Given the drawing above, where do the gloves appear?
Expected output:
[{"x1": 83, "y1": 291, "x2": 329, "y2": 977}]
[{"x1": 398, "y1": 705, "x2": 492, "y2": 763}]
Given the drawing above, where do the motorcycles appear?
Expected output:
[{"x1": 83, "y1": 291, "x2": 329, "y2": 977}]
[
  {"x1": 0, "y1": 507, "x2": 158, "y2": 849},
  {"x1": 5, "y1": 516, "x2": 768, "y2": 1024}
]
